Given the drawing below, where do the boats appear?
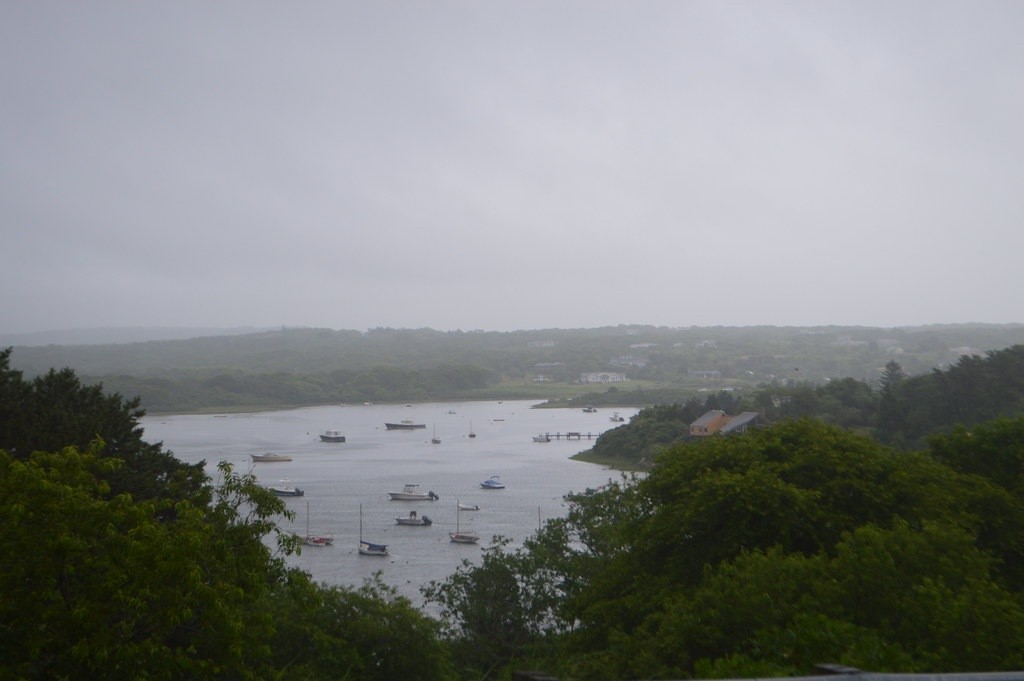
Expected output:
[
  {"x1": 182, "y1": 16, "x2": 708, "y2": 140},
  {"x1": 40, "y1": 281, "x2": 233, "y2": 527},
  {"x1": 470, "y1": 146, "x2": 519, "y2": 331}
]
[
  {"x1": 583, "y1": 408, "x2": 597, "y2": 413},
  {"x1": 609, "y1": 416, "x2": 625, "y2": 422},
  {"x1": 387, "y1": 484, "x2": 439, "y2": 501},
  {"x1": 386, "y1": 423, "x2": 425, "y2": 430},
  {"x1": 267, "y1": 487, "x2": 305, "y2": 497},
  {"x1": 468, "y1": 416, "x2": 476, "y2": 437},
  {"x1": 480, "y1": 479, "x2": 505, "y2": 489},
  {"x1": 449, "y1": 499, "x2": 479, "y2": 543},
  {"x1": 458, "y1": 504, "x2": 479, "y2": 511},
  {"x1": 250, "y1": 453, "x2": 291, "y2": 461},
  {"x1": 432, "y1": 423, "x2": 441, "y2": 443},
  {"x1": 299, "y1": 501, "x2": 333, "y2": 546},
  {"x1": 319, "y1": 429, "x2": 345, "y2": 443},
  {"x1": 358, "y1": 504, "x2": 388, "y2": 556},
  {"x1": 394, "y1": 516, "x2": 428, "y2": 526},
  {"x1": 532, "y1": 436, "x2": 550, "y2": 442}
]
